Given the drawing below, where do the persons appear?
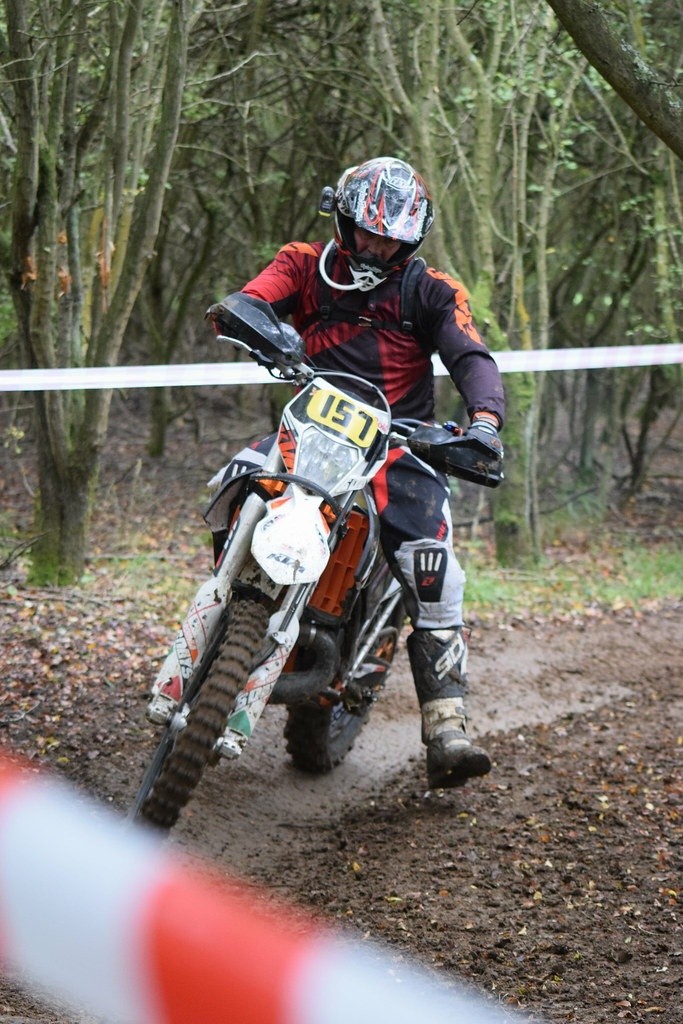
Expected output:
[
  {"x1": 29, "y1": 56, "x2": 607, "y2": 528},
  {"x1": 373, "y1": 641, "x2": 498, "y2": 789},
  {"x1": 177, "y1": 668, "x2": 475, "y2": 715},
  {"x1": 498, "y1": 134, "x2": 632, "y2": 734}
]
[{"x1": 210, "y1": 154, "x2": 506, "y2": 786}]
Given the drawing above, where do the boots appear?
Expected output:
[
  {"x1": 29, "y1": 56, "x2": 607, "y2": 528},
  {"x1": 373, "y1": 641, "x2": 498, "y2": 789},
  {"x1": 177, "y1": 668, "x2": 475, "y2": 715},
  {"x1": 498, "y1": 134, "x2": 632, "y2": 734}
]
[{"x1": 406, "y1": 629, "x2": 492, "y2": 788}]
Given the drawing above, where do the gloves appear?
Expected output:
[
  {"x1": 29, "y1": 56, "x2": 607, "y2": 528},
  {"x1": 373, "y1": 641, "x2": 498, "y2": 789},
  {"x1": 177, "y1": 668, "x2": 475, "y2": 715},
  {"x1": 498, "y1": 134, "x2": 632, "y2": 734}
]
[{"x1": 466, "y1": 420, "x2": 504, "y2": 461}]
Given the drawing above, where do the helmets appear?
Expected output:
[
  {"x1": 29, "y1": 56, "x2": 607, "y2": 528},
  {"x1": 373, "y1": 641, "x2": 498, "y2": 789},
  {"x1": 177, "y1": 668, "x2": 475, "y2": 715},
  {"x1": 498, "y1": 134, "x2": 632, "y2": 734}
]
[{"x1": 334, "y1": 157, "x2": 431, "y2": 279}]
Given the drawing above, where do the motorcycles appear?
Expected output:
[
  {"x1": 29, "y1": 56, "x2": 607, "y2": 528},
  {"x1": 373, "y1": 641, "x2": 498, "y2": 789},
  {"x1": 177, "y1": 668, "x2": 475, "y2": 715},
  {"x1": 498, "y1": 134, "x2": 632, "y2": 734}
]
[{"x1": 134, "y1": 291, "x2": 505, "y2": 839}]
